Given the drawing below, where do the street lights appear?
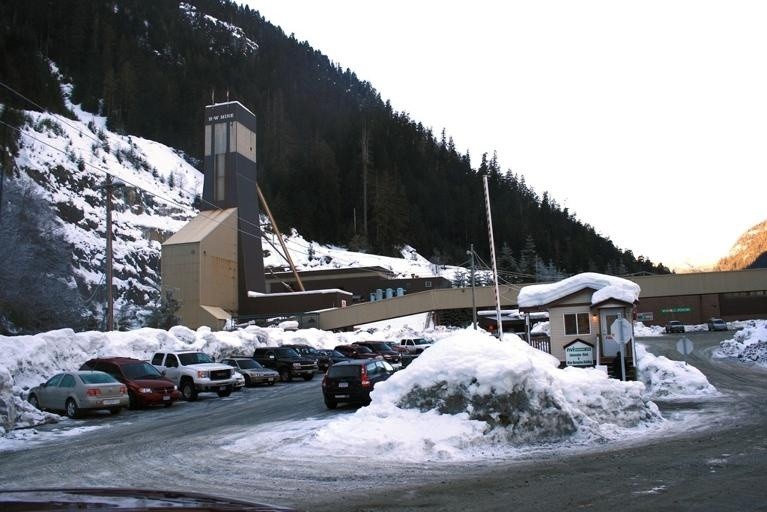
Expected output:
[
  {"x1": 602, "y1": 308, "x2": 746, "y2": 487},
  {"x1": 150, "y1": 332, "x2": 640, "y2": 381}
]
[
  {"x1": 465, "y1": 243, "x2": 477, "y2": 330},
  {"x1": 91, "y1": 170, "x2": 126, "y2": 332}
]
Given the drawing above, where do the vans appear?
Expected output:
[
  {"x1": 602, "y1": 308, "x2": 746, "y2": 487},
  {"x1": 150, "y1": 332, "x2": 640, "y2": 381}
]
[{"x1": 709, "y1": 319, "x2": 728, "y2": 331}]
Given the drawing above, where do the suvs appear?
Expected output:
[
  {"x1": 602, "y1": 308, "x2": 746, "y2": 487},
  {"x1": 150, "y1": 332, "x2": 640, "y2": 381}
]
[{"x1": 666, "y1": 320, "x2": 685, "y2": 334}]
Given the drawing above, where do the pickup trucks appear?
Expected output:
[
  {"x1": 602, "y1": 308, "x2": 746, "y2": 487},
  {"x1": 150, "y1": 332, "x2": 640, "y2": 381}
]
[{"x1": 151, "y1": 349, "x2": 236, "y2": 402}]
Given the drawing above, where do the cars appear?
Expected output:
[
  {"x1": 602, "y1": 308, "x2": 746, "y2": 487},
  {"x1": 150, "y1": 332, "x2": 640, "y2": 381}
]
[
  {"x1": 219, "y1": 335, "x2": 443, "y2": 411},
  {"x1": 77, "y1": 357, "x2": 178, "y2": 411},
  {"x1": 27, "y1": 370, "x2": 131, "y2": 418}
]
